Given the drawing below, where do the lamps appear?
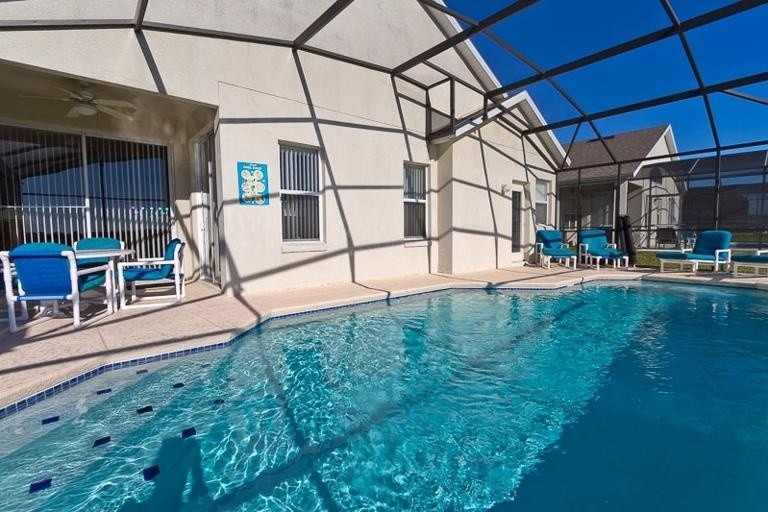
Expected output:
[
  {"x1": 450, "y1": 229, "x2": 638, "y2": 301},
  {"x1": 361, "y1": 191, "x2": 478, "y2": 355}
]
[{"x1": 73, "y1": 99, "x2": 98, "y2": 116}]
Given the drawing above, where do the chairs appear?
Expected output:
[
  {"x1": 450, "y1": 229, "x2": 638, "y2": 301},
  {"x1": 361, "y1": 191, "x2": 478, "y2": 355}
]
[
  {"x1": 534, "y1": 224, "x2": 577, "y2": 271},
  {"x1": 732, "y1": 250, "x2": 768, "y2": 277},
  {"x1": 578, "y1": 230, "x2": 629, "y2": 269},
  {"x1": 656, "y1": 230, "x2": 731, "y2": 273},
  {"x1": 0, "y1": 238, "x2": 185, "y2": 334}
]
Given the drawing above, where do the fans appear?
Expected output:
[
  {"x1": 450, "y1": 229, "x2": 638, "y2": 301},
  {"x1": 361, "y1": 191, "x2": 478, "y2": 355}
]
[{"x1": 17, "y1": 84, "x2": 136, "y2": 121}]
